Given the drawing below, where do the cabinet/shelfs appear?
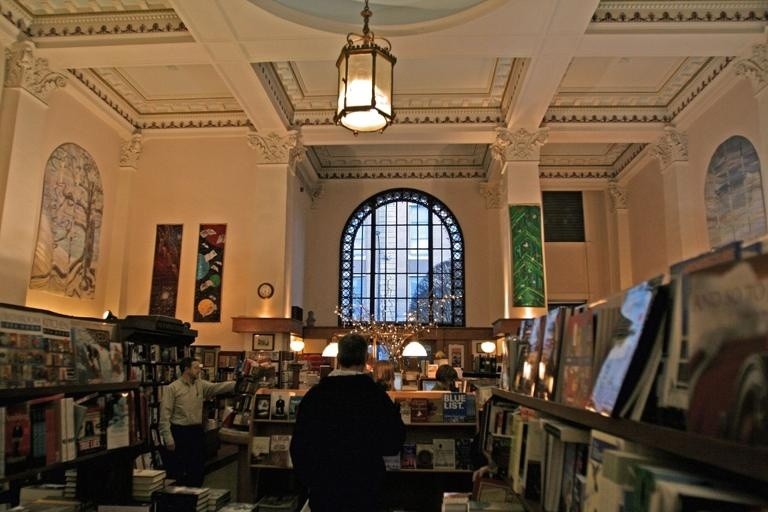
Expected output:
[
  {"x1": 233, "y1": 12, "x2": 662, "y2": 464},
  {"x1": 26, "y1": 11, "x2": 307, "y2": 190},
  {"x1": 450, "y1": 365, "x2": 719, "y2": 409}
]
[{"x1": 0, "y1": 242, "x2": 767, "y2": 512}]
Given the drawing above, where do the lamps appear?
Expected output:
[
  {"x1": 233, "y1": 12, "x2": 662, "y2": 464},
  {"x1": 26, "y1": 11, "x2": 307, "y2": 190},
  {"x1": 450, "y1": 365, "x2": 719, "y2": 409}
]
[{"x1": 332, "y1": 0, "x2": 400, "y2": 137}]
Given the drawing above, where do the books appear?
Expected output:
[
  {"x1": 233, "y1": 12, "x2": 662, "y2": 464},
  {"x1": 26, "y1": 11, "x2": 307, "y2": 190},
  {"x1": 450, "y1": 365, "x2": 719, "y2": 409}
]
[{"x1": 1, "y1": 239, "x2": 768, "y2": 512}]
